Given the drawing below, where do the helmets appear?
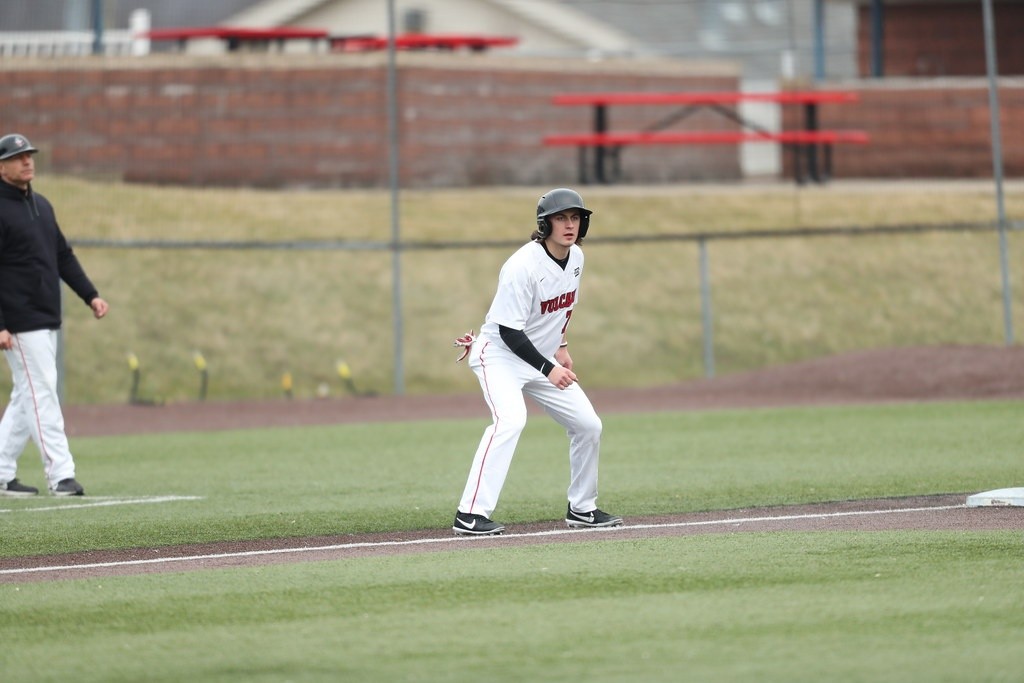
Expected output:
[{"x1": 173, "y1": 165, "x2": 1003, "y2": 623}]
[
  {"x1": 0, "y1": 134, "x2": 38, "y2": 161},
  {"x1": 537, "y1": 188, "x2": 593, "y2": 238}
]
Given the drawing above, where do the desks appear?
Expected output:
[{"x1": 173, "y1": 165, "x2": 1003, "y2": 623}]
[{"x1": 551, "y1": 91, "x2": 859, "y2": 183}]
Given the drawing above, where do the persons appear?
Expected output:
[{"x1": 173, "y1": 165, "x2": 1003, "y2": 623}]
[
  {"x1": 0, "y1": 134, "x2": 107, "y2": 497},
  {"x1": 452, "y1": 188, "x2": 624, "y2": 537}
]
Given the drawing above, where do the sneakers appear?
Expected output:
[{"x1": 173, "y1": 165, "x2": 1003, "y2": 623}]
[
  {"x1": 48, "y1": 478, "x2": 84, "y2": 496},
  {"x1": 452, "y1": 510, "x2": 505, "y2": 534},
  {"x1": 0, "y1": 478, "x2": 39, "y2": 496},
  {"x1": 565, "y1": 501, "x2": 623, "y2": 527}
]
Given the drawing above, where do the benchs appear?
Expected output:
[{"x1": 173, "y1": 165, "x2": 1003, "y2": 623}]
[
  {"x1": 134, "y1": 27, "x2": 328, "y2": 54},
  {"x1": 328, "y1": 32, "x2": 519, "y2": 52},
  {"x1": 543, "y1": 126, "x2": 869, "y2": 186}
]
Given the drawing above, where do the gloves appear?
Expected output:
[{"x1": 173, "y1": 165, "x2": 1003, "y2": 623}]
[{"x1": 454, "y1": 329, "x2": 477, "y2": 363}]
